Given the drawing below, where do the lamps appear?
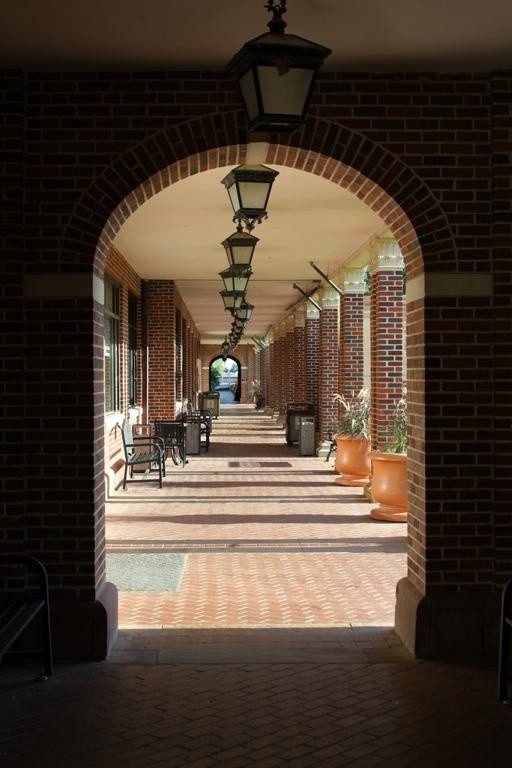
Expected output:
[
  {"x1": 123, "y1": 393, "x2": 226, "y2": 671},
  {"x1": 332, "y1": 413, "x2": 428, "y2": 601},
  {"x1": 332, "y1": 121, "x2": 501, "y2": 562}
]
[
  {"x1": 226, "y1": 0, "x2": 334, "y2": 131},
  {"x1": 220, "y1": 160, "x2": 279, "y2": 362}
]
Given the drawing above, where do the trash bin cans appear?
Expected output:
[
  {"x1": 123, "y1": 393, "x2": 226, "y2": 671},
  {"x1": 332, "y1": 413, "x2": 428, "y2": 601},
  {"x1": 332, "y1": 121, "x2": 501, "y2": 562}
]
[
  {"x1": 201, "y1": 391, "x2": 220, "y2": 420},
  {"x1": 286, "y1": 401, "x2": 315, "y2": 447}
]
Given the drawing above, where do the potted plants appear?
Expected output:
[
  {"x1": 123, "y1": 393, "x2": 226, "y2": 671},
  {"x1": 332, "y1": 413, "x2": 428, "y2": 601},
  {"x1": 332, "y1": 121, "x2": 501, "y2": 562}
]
[{"x1": 331, "y1": 385, "x2": 410, "y2": 523}]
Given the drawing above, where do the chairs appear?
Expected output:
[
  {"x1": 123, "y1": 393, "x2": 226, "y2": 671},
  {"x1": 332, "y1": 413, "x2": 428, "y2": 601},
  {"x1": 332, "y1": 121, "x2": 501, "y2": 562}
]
[
  {"x1": 117, "y1": 410, "x2": 164, "y2": 490},
  {"x1": 181, "y1": 402, "x2": 212, "y2": 451}
]
